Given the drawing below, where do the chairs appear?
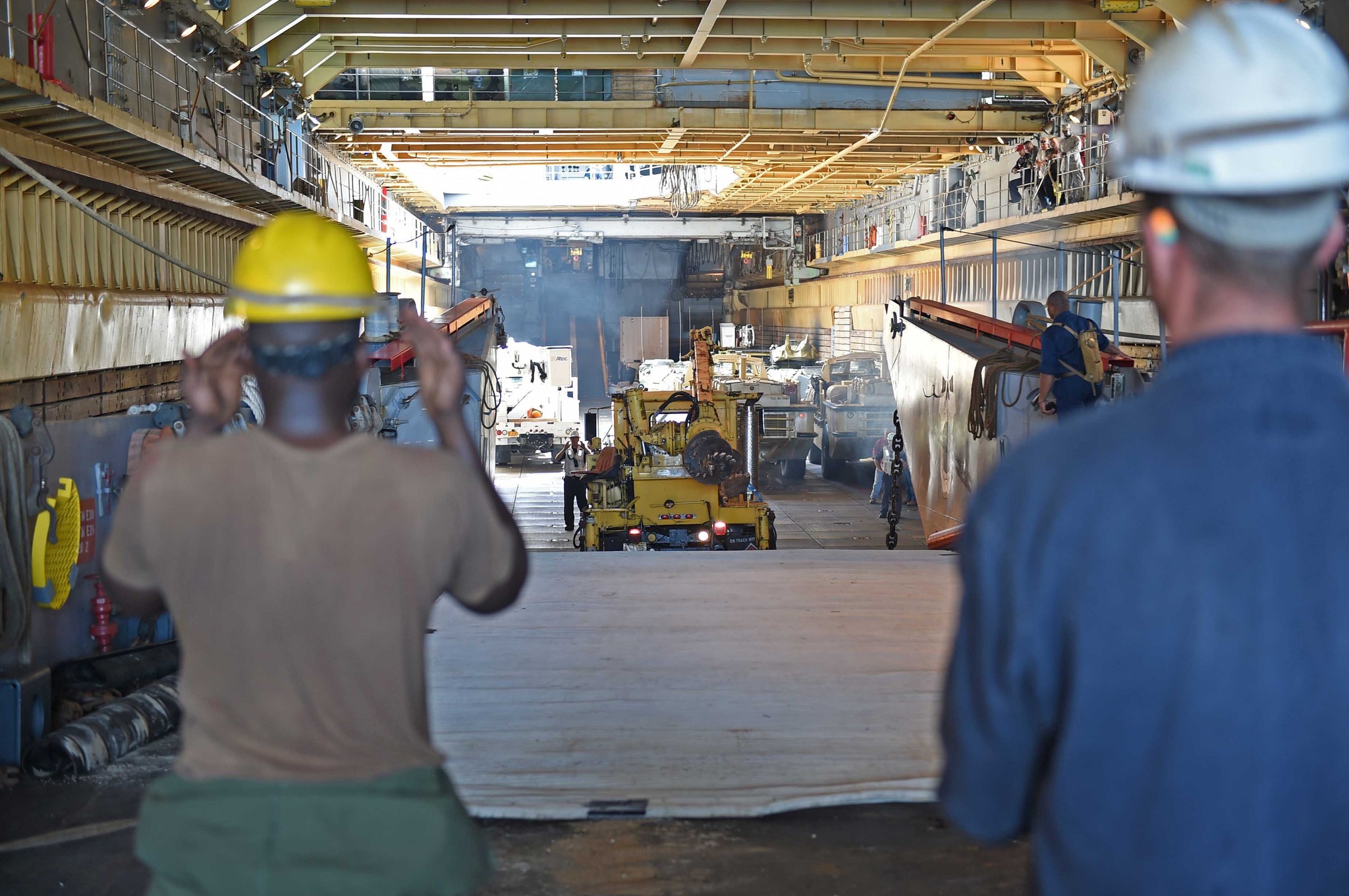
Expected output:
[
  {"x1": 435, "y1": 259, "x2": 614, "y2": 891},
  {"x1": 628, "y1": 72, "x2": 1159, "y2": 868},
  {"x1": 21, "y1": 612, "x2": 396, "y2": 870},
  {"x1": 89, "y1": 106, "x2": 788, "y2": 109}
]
[{"x1": 570, "y1": 446, "x2": 616, "y2": 477}]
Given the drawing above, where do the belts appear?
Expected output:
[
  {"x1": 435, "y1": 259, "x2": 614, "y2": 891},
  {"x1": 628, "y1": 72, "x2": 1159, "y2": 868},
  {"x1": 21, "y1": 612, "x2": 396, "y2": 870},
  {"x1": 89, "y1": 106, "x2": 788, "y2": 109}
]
[
  {"x1": 1061, "y1": 371, "x2": 1085, "y2": 377},
  {"x1": 884, "y1": 472, "x2": 894, "y2": 476}
]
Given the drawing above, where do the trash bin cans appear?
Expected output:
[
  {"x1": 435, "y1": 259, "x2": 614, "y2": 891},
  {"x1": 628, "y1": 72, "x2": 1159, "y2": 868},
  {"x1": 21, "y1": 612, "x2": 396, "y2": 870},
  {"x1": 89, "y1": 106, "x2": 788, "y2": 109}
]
[{"x1": 720, "y1": 322, "x2": 736, "y2": 348}]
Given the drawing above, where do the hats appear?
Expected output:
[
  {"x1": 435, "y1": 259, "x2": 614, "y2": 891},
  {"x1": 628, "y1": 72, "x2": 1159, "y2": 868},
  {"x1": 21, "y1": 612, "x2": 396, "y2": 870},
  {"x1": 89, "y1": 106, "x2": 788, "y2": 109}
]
[
  {"x1": 887, "y1": 432, "x2": 896, "y2": 440},
  {"x1": 1015, "y1": 144, "x2": 1024, "y2": 151},
  {"x1": 1061, "y1": 124, "x2": 1070, "y2": 131}
]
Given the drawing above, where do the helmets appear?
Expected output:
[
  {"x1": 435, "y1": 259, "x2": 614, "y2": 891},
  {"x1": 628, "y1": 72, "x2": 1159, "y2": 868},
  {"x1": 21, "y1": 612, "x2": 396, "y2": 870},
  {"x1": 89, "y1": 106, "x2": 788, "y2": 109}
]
[
  {"x1": 568, "y1": 430, "x2": 581, "y2": 438},
  {"x1": 222, "y1": 211, "x2": 371, "y2": 321},
  {"x1": 1106, "y1": 1, "x2": 1348, "y2": 251}
]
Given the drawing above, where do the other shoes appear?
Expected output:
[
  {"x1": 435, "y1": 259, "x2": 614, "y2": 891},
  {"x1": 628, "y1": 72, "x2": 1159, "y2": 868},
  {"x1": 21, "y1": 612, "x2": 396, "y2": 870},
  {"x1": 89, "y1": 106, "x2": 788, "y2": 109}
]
[
  {"x1": 1047, "y1": 204, "x2": 1056, "y2": 209},
  {"x1": 1066, "y1": 199, "x2": 1073, "y2": 203},
  {"x1": 869, "y1": 498, "x2": 876, "y2": 503},
  {"x1": 1008, "y1": 198, "x2": 1021, "y2": 204},
  {"x1": 907, "y1": 499, "x2": 917, "y2": 505},
  {"x1": 1078, "y1": 198, "x2": 1084, "y2": 202},
  {"x1": 565, "y1": 526, "x2": 574, "y2": 530},
  {"x1": 1033, "y1": 207, "x2": 1046, "y2": 214},
  {"x1": 879, "y1": 514, "x2": 884, "y2": 517},
  {"x1": 902, "y1": 499, "x2": 910, "y2": 503}
]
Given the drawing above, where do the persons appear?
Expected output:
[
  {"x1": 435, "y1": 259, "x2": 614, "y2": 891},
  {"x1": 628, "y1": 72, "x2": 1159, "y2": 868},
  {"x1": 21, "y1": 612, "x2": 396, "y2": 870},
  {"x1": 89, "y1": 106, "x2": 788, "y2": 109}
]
[
  {"x1": 870, "y1": 429, "x2": 917, "y2": 518},
  {"x1": 100, "y1": 210, "x2": 527, "y2": 896},
  {"x1": 555, "y1": 430, "x2": 589, "y2": 530},
  {"x1": 935, "y1": 0, "x2": 1349, "y2": 896},
  {"x1": 1039, "y1": 291, "x2": 1133, "y2": 427},
  {"x1": 1008, "y1": 123, "x2": 1085, "y2": 214}
]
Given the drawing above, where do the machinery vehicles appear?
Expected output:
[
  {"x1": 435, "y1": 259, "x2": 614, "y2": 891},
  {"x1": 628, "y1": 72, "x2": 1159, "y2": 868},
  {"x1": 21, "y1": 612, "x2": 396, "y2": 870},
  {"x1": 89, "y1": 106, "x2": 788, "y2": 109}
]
[
  {"x1": 636, "y1": 323, "x2": 904, "y2": 481},
  {"x1": 572, "y1": 327, "x2": 778, "y2": 551},
  {"x1": 493, "y1": 342, "x2": 581, "y2": 465}
]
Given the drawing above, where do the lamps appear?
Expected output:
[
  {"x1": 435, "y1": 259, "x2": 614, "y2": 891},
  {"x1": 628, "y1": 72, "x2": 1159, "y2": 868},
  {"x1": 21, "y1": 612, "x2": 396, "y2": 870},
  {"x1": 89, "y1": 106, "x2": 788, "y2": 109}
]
[
  {"x1": 975, "y1": 94, "x2": 1119, "y2": 153},
  {"x1": 134, "y1": 0, "x2": 321, "y2": 132}
]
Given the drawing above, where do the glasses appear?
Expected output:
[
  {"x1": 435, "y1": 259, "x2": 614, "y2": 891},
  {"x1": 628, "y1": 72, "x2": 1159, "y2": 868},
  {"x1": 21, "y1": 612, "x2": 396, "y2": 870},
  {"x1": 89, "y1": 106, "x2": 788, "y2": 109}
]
[{"x1": 1040, "y1": 140, "x2": 1046, "y2": 143}]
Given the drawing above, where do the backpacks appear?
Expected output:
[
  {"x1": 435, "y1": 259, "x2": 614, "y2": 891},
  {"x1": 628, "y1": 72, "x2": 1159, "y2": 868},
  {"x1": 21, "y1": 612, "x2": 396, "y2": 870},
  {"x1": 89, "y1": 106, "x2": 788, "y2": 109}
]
[{"x1": 1053, "y1": 318, "x2": 1105, "y2": 383}]
